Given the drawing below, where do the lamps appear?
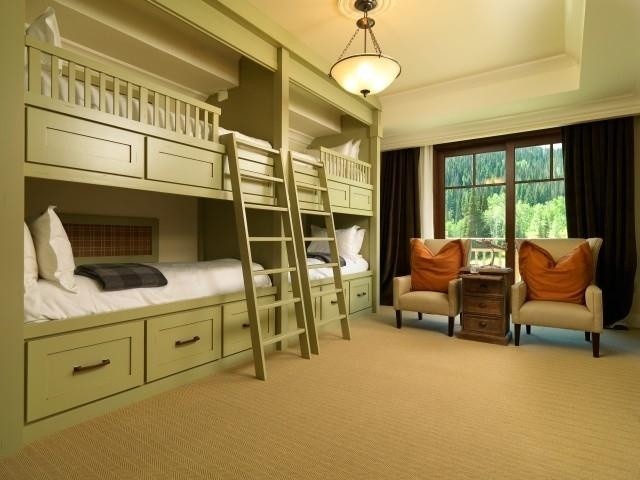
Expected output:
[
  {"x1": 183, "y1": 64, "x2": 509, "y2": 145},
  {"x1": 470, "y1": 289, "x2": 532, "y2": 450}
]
[{"x1": 328, "y1": 0, "x2": 401, "y2": 98}]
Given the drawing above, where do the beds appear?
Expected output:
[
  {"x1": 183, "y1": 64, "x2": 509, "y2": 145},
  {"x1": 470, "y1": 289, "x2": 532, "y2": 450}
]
[{"x1": 0, "y1": 0, "x2": 383, "y2": 457}]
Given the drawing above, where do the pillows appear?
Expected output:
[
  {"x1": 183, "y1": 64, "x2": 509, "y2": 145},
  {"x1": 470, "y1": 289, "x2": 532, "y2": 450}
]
[
  {"x1": 519, "y1": 240, "x2": 594, "y2": 304},
  {"x1": 410, "y1": 238, "x2": 466, "y2": 294}
]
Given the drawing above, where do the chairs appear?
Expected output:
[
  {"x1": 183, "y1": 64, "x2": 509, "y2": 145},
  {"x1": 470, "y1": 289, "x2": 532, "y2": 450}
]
[
  {"x1": 393, "y1": 238, "x2": 472, "y2": 337},
  {"x1": 511, "y1": 238, "x2": 603, "y2": 358}
]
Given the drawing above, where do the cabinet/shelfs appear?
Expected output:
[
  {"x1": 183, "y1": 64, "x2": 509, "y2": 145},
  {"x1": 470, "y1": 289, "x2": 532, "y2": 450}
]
[{"x1": 456, "y1": 273, "x2": 512, "y2": 346}]
